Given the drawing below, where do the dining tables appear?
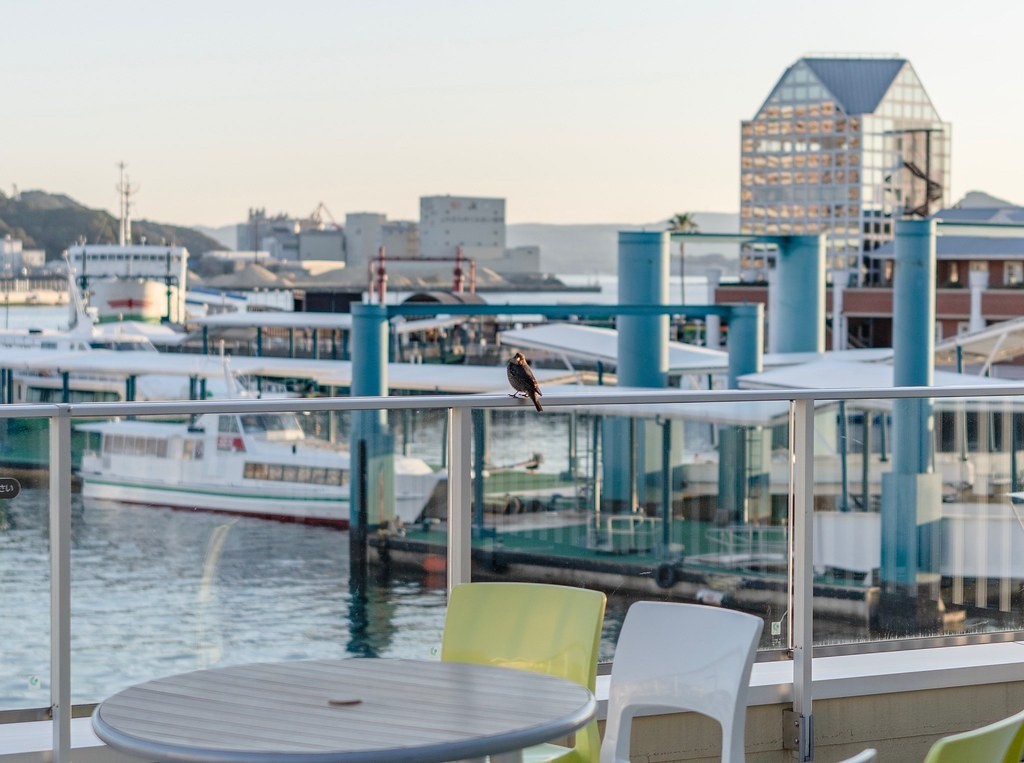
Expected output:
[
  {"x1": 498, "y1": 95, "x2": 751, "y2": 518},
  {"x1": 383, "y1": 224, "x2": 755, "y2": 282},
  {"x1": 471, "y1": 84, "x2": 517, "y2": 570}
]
[{"x1": 89, "y1": 658, "x2": 597, "y2": 763}]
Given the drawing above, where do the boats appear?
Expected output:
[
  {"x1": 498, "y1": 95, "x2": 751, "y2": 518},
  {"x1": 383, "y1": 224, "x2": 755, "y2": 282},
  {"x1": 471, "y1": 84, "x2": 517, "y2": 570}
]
[{"x1": 0, "y1": 155, "x2": 1022, "y2": 640}]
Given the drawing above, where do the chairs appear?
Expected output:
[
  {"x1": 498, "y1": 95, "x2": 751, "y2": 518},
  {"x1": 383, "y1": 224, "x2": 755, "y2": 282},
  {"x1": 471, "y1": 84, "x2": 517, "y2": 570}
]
[
  {"x1": 438, "y1": 583, "x2": 603, "y2": 763},
  {"x1": 923, "y1": 708, "x2": 1022, "y2": 763},
  {"x1": 591, "y1": 601, "x2": 764, "y2": 763}
]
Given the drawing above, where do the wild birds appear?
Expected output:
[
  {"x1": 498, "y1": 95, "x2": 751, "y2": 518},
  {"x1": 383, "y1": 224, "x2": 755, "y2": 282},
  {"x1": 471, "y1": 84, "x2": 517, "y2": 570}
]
[{"x1": 506, "y1": 352, "x2": 544, "y2": 413}]
